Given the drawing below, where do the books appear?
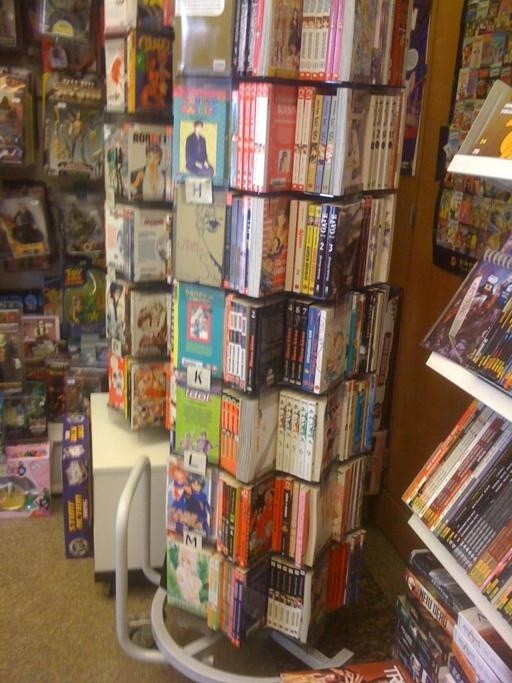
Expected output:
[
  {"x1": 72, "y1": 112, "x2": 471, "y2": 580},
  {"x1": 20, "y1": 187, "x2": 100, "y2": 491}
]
[{"x1": 104, "y1": 1, "x2": 512, "y2": 683}]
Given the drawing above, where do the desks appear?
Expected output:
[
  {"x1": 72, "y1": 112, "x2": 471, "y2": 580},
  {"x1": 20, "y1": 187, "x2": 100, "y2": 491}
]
[{"x1": 89, "y1": 391, "x2": 169, "y2": 598}]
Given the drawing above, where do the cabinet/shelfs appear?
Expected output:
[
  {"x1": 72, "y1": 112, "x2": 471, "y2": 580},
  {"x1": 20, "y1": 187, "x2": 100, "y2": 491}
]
[{"x1": 407, "y1": 151, "x2": 512, "y2": 652}]
